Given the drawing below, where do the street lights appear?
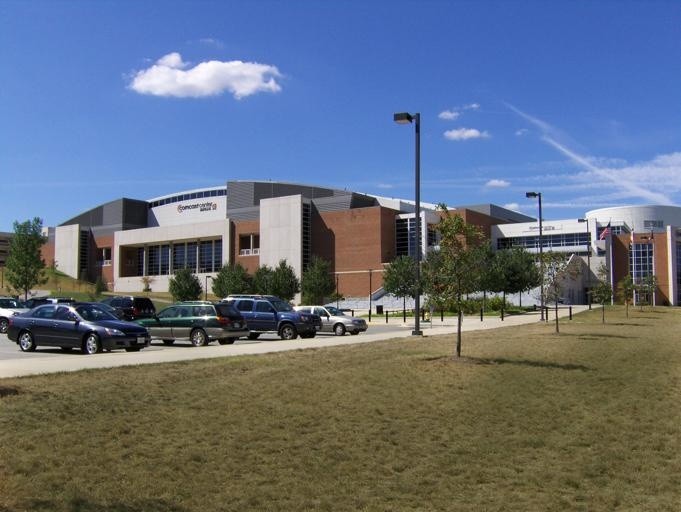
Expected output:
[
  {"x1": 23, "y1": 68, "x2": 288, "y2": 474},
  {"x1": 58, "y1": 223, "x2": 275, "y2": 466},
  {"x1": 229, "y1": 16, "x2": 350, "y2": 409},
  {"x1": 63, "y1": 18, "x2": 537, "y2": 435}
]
[
  {"x1": 205, "y1": 275, "x2": 212, "y2": 300},
  {"x1": 525, "y1": 191, "x2": 546, "y2": 321},
  {"x1": 578, "y1": 218, "x2": 593, "y2": 310},
  {"x1": 393, "y1": 112, "x2": 424, "y2": 331}
]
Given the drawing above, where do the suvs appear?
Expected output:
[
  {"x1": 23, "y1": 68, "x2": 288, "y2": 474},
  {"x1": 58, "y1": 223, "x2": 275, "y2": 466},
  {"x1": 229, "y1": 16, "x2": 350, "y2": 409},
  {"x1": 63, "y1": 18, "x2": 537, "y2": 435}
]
[
  {"x1": 100, "y1": 297, "x2": 156, "y2": 322},
  {"x1": 222, "y1": 297, "x2": 324, "y2": 340},
  {"x1": 131, "y1": 301, "x2": 250, "y2": 346}
]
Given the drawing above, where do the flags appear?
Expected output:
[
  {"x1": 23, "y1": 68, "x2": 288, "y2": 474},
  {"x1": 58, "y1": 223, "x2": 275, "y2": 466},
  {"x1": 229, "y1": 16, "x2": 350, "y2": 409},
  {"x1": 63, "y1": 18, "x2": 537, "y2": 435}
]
[{"x1": 598, "y1": 221, "x2": 611, "y2": 240}]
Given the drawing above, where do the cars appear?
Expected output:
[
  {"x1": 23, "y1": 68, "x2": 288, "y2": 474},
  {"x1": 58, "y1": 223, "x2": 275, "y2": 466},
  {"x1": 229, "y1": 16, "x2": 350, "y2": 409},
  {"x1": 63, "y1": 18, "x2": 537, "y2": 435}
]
[
  {"x1": 1, "y1": 297, "x2": 35, "y2": 334},
  {"x1": 6, "y1": 302, "x2": 151, "y2": 354},
  {"x1": 25, "y1": 297, "x2": 77, "y2": 313},
  {"x1": 291, "y1": 306, "x2": 368, "y2": 336},
  {"x1": 72, "y1": 302, "x2": 124, "y2": 321}
]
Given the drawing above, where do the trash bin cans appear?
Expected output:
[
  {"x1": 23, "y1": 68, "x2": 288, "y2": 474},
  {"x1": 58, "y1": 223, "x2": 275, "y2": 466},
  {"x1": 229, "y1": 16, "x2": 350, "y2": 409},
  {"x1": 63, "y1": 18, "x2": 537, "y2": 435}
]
[{"x1": 377, "y1": 305, "x2": 383, "y2": 315}]
